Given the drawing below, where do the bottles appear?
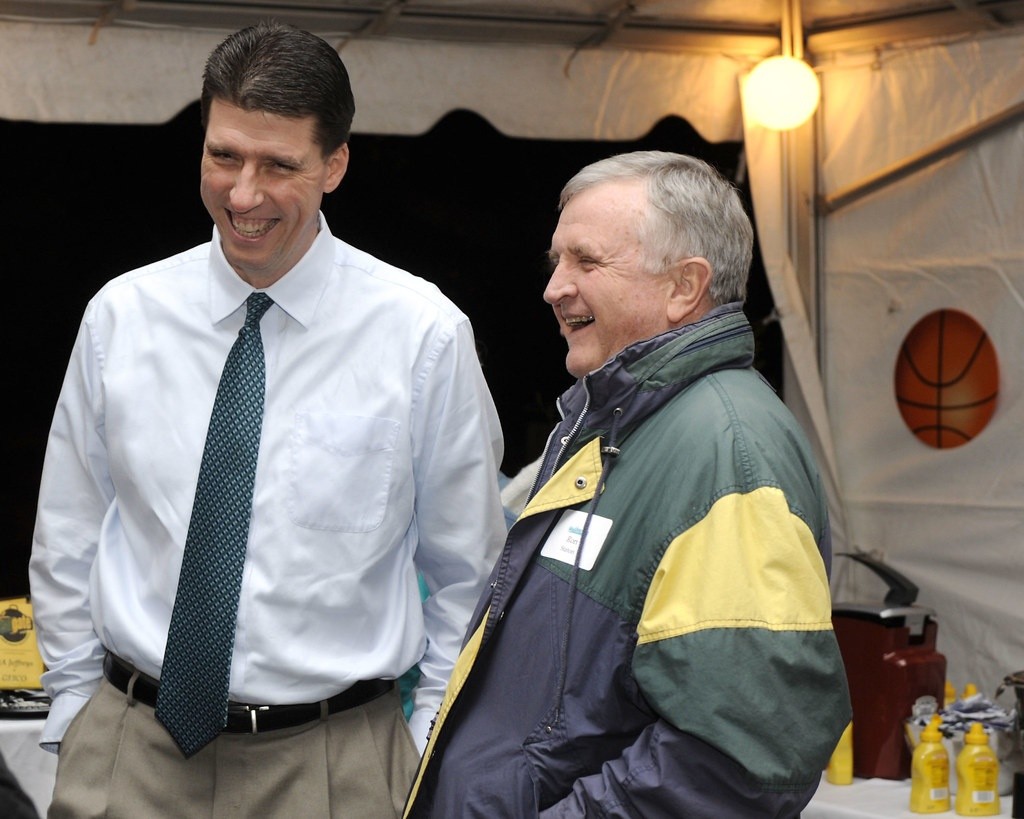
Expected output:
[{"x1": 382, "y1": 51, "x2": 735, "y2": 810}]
[
  {"x1": 828, "y1": 720, "x2": 853, "y2": 785},
  {"x1": 909, "y1": 714, "x2": 951, "y2": 813},
  {"x1": 955, "y1": 724, "x2": 1000, "y2": 815},
  {"x1": 944, "y1": 679, "x2": 976, "y2": 708}
]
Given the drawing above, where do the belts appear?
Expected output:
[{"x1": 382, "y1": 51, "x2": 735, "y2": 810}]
[{"x1": 102, "y1": 650, "x2": 394, "y2": 735}]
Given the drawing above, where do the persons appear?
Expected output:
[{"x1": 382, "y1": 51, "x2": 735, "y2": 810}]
[
  {"x1": 25, "y1": 15, "x2": 511, "y2": 819},
  {"x1": 394, "y1": 144, "x2": 855, "y2": 819}
]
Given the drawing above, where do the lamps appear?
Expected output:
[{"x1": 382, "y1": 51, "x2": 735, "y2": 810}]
[{"x1": 743, "y1": 0, "x2": 822, "y2": 134}]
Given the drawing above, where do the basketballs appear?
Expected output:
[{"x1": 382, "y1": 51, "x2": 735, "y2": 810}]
[{"x1": 894, "y1": 308, "x2": 999, "y2": 450}]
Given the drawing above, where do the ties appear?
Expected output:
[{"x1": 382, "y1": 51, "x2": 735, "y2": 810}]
[{"x1": 154, "y1": 293, "x2": 277, "y2": 759}]
